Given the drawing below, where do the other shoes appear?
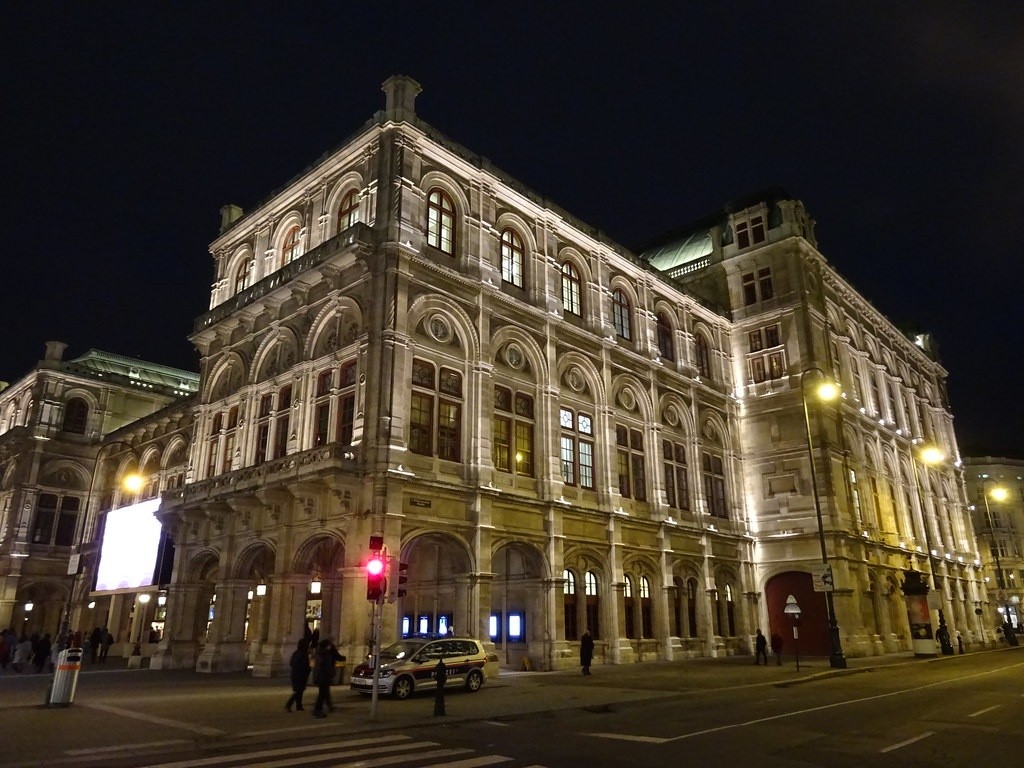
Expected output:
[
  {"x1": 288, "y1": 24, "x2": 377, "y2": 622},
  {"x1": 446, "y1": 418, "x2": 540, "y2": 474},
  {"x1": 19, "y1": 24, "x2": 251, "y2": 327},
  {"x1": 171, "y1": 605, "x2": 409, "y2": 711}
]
[
  {"x1": 313, "y1": 711, "x2": 327, "y2": 718},
  {"x1": 297, "y1": 707, "x2": 304, "y2": 711},
  {"x1": 285, "y1": 705, "x2": 293, "y2": 713},
  {"x1": 582, "y1": 668, "x2": 592, "y2": 676}
]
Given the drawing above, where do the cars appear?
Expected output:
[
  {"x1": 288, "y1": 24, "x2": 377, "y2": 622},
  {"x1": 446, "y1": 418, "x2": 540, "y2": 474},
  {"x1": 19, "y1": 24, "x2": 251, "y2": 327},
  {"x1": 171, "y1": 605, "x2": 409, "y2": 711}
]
[{"x1": 351, "y1": 631, "x2": 487, "y2": 700}]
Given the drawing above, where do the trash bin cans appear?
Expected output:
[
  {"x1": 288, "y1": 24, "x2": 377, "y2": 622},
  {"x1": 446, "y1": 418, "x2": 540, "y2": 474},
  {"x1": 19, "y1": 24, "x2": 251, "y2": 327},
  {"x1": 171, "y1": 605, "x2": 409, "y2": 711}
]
[{"x1": 47, "y1": 647, "x2": 83, "y2": 708}]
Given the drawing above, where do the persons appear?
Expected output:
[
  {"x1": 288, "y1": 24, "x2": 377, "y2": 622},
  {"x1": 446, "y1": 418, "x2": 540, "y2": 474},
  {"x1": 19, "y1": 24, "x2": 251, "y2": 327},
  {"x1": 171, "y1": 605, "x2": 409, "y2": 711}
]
[
  {"x1": 580, "y1": 628, "x2": 595, "y2": 675},
  {"x1": 127, "y1": 630, "x2": 131, "y2": 642},
  {"x1": 753, "y1": 629, "x2": 767, "y2": 665},
  {"x1": 771, "y1": 630, "x2": 784, "y2": 666},
  {"x1": 149, "y1": 626, "x2": 161, "y2": 643},
  {"x1": 0, "y1": 627, "x2": 114, "y2": 673},
  {"x1": 284, "y1": 622, "x2": 346, "y2": 717}
]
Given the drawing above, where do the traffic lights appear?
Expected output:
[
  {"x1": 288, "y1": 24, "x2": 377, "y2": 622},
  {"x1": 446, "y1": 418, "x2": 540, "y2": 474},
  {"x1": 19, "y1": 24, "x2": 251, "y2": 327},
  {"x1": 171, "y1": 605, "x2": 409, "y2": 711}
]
[
  {"x1": 366, "y1": 558, "x2": 381, "y2": 601},
  {"x1": 388, "y1": 558, "x2": 409, "y2": 604}
]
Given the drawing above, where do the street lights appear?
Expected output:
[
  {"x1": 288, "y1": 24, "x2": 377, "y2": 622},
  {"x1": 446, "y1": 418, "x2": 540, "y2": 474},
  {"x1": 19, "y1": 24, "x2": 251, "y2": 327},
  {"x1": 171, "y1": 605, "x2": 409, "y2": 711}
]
[
  {"x1": 56, "y1": 440, "x2": 145, "y2": 654},
  {"x1": 800, "y1": 367, "x2": 849, "y2": 671},
  {"x1": 20, "y1": 599, "x2": 34, "y2": 640},
  {"x1": 981, "y1": 477, "x2": 1014, "y2": 629},
  {"x1": 909, "y1": 436, "x2": 955, "y2": 655}
]
